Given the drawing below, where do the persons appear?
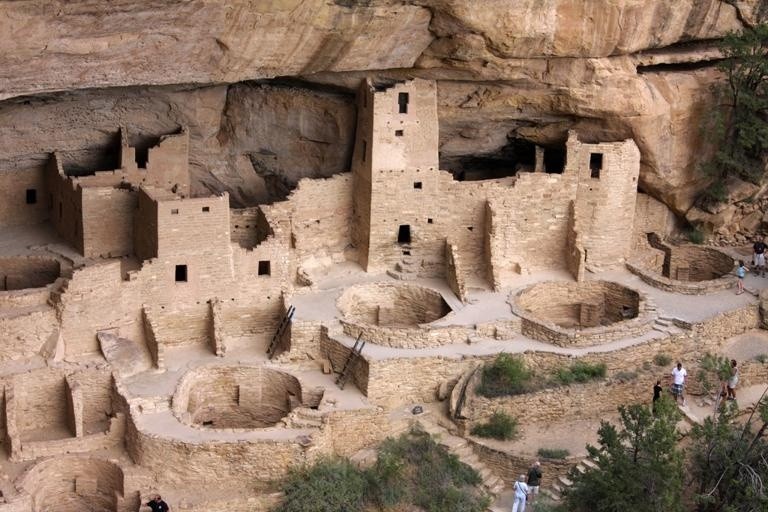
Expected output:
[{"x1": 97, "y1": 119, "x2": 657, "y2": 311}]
[
  {"x1": 735, "y1": 237, "x2": 768, "y2": 295},
  {"x1": 664, "y1": 363, "x2": 688, "y2": 407},
  {"x1": 726, "y1": 360, "x2": 738, "y2": 401},
  {"x1": 511, "y1": 461, "x2": 542, "y2": 512},
  {"x1": 142, "y1": 494, "x2": 170, "y2": 512},
  {"x1": 718, "y1": 386, "x2": 727, "y2": 400},
  {"x1": 653, "y1": 381, "x2": 663, "y2": 419}
]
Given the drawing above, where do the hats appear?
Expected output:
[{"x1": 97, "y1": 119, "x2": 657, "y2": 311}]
[{"x1": 519, "y1": 475, "x2": 525, "y2": 482}]
